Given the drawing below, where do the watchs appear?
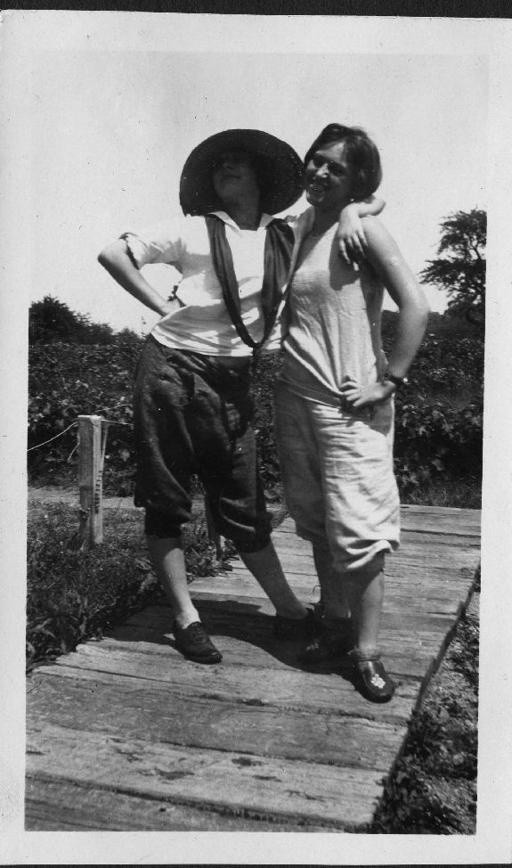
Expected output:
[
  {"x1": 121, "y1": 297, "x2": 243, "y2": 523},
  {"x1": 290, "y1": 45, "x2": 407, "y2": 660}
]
[{"x1": 383, "y1": 372, "x2": 402, "y2": 385}]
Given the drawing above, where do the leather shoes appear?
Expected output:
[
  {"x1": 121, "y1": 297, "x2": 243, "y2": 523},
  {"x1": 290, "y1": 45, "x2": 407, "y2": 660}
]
[
  {"x1": 348, "y1": 658, "x2": 395, "y2": 703},
  {"x1": 272, "y1": 608, "x2": 354, "y2": 661},
  {"x1": 169, "y1": 621, "x2": 222, "y2": 664}
]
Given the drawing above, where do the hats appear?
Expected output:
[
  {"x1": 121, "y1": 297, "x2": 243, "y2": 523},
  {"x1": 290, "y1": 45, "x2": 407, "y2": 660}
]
[{"x1": 179, "y1": 128, "x2": 310, "y2": 215}]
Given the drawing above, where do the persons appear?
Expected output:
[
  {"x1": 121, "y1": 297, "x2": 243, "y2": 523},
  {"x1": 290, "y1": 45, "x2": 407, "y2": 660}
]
[
  {"x1": 276, "y1": 123, "x2": 428, "y2": 703},
  {"x1": 98, "y1": 129, "x2": 384, "y2": 664}
]
